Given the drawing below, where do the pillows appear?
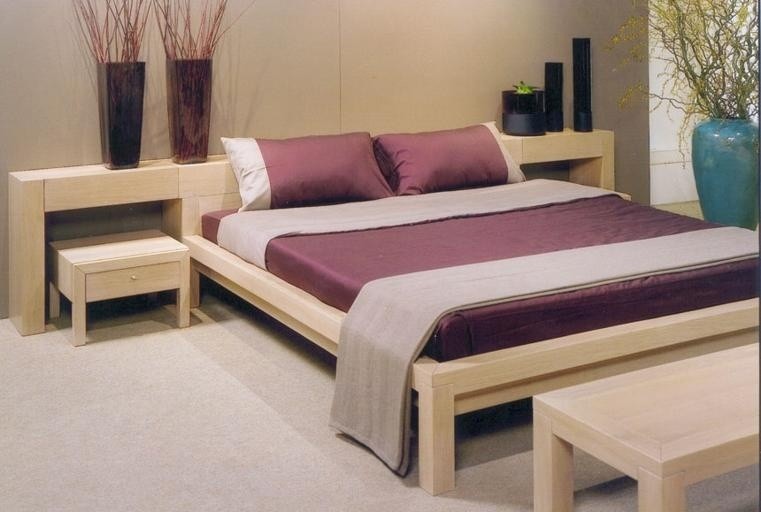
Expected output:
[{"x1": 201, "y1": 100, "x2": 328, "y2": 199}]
[
  {"x1": 220, "y1": 133, "x2": 395, "y2": 211},
  {"x1": 372, "y1": 123, "x2": 525, "y2": 195}
]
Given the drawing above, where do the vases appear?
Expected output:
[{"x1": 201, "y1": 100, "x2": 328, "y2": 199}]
[
  {"x1": 165, "y1": 60, "x2": 210, "y2": 163},
  {"x1": 96, "y1": 62, "x2": 145, "y2": 169}
]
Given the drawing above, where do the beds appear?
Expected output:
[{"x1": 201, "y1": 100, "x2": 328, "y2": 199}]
[{"x1": 8, "y1": 128, "x2": 761, "y2": 496}]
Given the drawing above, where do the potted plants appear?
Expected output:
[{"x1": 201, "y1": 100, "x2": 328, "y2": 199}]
[
  {"x1": 617, "y1": 0, "x2": 761, "y2": 230},
  {"x1": 502, "y1": 80, "x2": 548, "y2": 136}
]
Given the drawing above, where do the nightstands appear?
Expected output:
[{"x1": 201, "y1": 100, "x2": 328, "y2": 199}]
[{"x1": 49, "y1": 228, "x2": 192, "y2": 347}]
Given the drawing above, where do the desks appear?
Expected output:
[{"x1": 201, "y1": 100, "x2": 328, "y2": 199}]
[{"x1": 533, "y1": 342, "x2": 761, "y2": 512}]
